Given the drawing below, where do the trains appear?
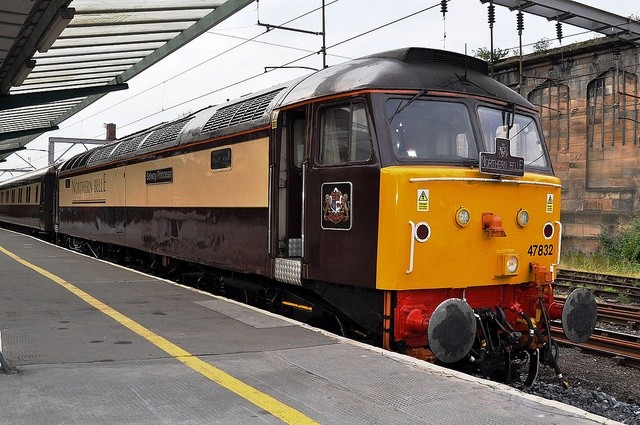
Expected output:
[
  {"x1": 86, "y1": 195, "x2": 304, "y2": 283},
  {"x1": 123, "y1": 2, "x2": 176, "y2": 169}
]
[{"x1": 1, "y1": 47, "x2": 599, "y2": 395}]
